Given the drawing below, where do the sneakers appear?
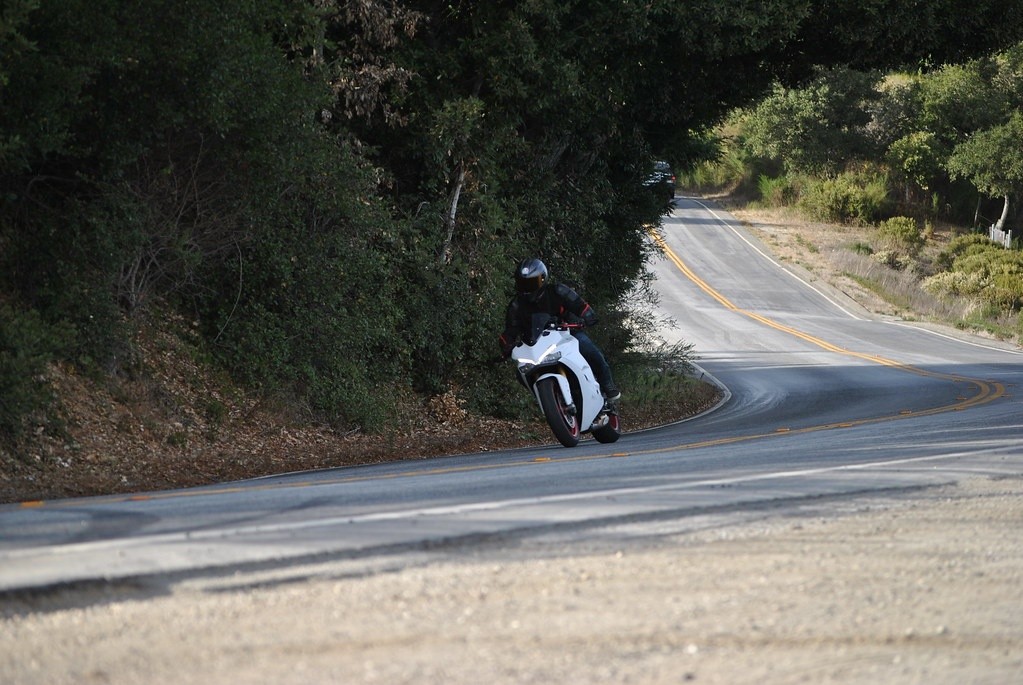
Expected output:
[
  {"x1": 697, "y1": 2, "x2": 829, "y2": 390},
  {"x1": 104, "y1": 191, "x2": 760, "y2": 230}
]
[{"x1": 604, "y1": 384, "x2": 621, "y2": 400}]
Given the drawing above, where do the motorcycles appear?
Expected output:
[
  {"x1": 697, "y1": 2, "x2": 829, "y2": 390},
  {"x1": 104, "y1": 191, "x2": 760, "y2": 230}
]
[{"x1": 494, "y1": 287, "x2": 621, "y2": 447}]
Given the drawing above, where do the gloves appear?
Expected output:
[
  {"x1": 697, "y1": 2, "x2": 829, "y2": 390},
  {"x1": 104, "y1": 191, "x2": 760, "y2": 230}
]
[
  {"x1": 585, "y1": 308, "x2": 600, "y2": 326},
  {"x1": 498, "y1": 336, "x2": 514, "y2": 356}
]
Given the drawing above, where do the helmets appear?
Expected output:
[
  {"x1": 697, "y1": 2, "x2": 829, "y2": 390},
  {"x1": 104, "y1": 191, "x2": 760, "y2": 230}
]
[{"x1": 516, "y1": 257, "x2": 548, "y2": 304}]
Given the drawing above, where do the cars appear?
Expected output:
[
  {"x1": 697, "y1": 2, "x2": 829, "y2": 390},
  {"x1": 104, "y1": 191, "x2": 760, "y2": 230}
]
[{"x1": 641, "y1": 159, "x2": 677, "y2": 198}]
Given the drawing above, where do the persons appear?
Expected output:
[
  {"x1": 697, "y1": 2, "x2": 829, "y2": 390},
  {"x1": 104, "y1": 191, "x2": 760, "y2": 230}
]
[{"x1": 505, "y1": 259, "x2": 621, "y2": 402}]
